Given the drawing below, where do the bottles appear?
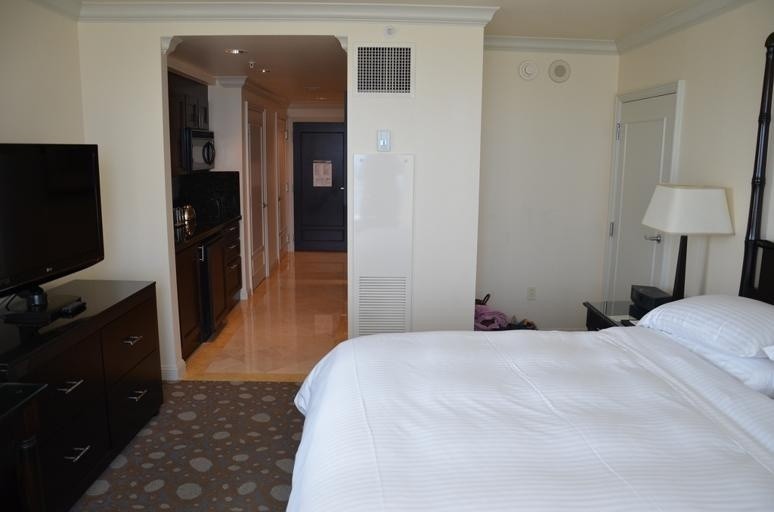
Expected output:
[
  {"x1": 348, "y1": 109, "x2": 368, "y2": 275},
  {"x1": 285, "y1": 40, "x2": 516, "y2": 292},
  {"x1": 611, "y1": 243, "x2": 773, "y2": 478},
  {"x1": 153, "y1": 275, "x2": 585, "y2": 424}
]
[
  {"x1": 185, "y1": 223, "x2": 195, "y2": 236},
  {"x1": 182, "y1": 206, "x2": 195, "y2": 220}
]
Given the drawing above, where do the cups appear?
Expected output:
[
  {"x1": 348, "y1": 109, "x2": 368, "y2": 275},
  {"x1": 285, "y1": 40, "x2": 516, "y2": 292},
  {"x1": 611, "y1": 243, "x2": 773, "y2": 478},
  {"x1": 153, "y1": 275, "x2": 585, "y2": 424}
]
[
  {"x1": 175, "y1": 226, "x2": 184, "y2": 244},
  {"x1": 173, "y1": 208, "x2": 183, "y2": 224}
]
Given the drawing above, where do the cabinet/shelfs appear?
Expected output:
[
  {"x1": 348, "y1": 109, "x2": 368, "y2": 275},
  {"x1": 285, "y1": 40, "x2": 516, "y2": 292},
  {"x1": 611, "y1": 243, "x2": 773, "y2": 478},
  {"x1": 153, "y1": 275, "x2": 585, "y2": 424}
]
[
  {"x1": 174, "y1": 213, "x2": 243, "y2": 362},
  {"x1": 167, "y1": 72, "x2": 209, "y2": 184},
  {"x1": 206, "y1": 85, "x2": 290, "y2": 301},
  {"x1": 0, "y1": 277, "x2": 164, "y2": 511}
]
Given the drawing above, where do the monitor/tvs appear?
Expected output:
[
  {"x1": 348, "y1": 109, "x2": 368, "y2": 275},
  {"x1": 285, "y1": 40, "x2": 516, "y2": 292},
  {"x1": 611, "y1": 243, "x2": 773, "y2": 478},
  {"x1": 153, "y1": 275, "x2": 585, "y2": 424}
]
[{"x1": 0, "y1": 144, "x2": 105, "y2": 323}]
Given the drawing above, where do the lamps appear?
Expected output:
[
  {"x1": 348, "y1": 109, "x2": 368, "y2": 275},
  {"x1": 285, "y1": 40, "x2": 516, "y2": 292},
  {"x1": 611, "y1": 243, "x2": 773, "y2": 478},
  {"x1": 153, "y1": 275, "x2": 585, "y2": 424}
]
[{"x1": 639, "y1": 185, "x2": 736, "y2": 299}]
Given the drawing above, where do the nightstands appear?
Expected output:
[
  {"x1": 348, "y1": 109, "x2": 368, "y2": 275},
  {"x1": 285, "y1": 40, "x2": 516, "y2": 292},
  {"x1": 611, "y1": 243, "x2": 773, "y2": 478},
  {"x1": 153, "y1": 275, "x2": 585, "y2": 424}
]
[{"x1": 580, "y1": 299, "x2": 638, "y2": 330}]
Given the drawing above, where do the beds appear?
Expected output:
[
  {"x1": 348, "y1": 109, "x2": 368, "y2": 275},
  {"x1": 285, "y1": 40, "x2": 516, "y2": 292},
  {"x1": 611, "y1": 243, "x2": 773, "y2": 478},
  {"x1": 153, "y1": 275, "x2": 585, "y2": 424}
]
[{"x1": 312, "y1": 238, "x2": 774, "y2": 512}]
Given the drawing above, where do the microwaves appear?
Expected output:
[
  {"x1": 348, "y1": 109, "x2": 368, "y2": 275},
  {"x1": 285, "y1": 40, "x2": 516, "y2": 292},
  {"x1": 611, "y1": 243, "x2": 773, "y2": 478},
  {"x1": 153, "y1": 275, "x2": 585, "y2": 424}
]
[{"x1": 181, "y1": 127, "x2": 215, "y2": 171}]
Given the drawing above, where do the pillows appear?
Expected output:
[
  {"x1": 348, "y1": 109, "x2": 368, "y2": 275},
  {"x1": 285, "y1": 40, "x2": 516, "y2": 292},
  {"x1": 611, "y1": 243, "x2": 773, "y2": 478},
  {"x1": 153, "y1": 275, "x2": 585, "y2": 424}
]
[{"x1": 637, "y1": 295, "x2": 773, "y2": 398}]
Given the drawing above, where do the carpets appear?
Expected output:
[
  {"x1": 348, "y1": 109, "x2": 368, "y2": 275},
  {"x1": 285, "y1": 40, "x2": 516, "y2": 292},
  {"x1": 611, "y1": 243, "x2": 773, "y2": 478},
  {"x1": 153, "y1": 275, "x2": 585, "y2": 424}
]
[{"x1": 70, "y1": 380, "x2": 305, "y2": 512}]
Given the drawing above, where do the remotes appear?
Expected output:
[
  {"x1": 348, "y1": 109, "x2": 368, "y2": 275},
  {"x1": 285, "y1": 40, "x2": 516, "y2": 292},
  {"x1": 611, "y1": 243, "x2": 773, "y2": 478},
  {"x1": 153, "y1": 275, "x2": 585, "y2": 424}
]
[{"x1": 62, "y1": 301, "x2": 86, "y2": 314}]
